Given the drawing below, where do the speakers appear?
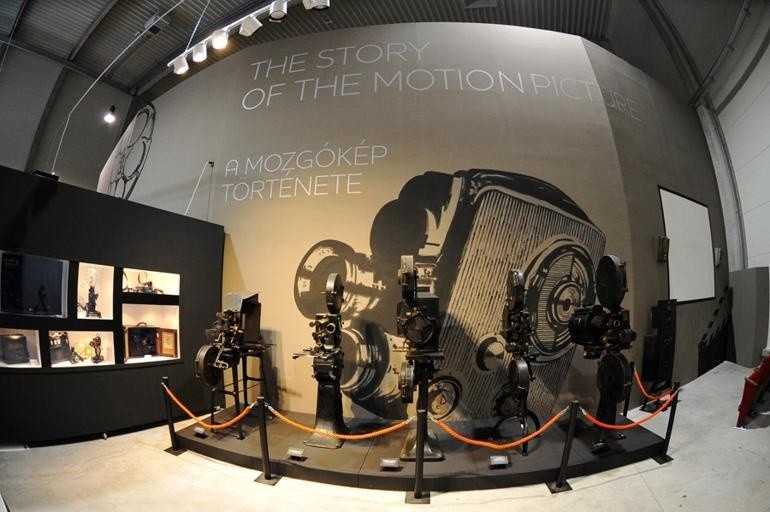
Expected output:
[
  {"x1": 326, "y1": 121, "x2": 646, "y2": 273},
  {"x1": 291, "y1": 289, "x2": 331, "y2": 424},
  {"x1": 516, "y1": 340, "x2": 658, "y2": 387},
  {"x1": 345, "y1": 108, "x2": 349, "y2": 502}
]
[
  {"x1": 714, "y1": 247, "x2": 724, "y2": 267},
  {"x1": 656, "y1": 234, "x2": 670, "y2": 261}
]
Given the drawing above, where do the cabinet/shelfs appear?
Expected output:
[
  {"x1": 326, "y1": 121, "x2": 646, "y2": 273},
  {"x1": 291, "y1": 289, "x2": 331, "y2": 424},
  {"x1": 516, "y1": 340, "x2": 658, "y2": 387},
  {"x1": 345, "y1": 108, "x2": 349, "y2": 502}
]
[{"x1": 0, "y1": 245, "x2": 184, "y2": 372}]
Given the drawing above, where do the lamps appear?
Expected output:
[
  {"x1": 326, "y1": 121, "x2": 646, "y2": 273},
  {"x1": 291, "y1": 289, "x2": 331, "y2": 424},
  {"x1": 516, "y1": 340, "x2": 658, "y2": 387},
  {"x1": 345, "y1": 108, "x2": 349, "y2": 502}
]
[{"x1": 165, "y1": 0, "x2": 331, "y2": 76}]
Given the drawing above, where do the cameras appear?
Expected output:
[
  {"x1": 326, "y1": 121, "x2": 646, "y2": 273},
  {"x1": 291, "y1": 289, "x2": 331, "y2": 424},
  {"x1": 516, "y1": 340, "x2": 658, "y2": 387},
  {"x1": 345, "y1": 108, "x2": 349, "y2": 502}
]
[{"x1": 294, "y1": 167, "x2": 606, "y2": 424}]
